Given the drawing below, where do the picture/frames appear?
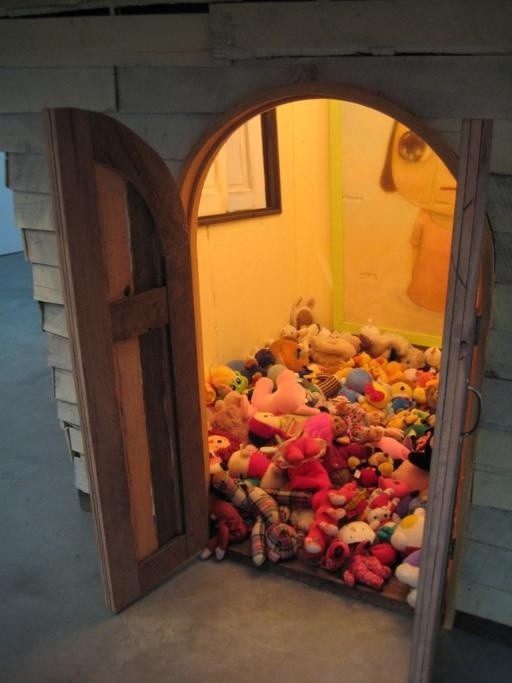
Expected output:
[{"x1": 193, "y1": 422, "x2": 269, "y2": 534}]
[{"x1": 174, "y1": 108, "x2": 282, "y2": 226}]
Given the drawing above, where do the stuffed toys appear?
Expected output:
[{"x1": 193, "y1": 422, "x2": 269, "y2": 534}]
[{"x1": 200, "y1": 295, "x2": 442, "y2": 609}]
